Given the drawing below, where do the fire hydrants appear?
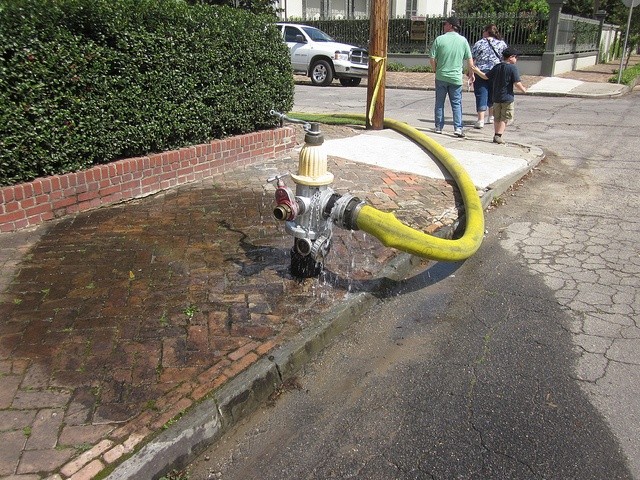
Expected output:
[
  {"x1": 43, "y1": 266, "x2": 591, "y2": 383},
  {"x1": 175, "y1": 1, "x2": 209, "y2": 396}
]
[{"x1": 267, "y1": 109, "x2": 366, "y2": 278}]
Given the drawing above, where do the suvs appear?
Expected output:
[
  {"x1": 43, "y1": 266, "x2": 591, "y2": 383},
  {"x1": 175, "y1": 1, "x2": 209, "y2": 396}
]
[{"x1": 263, "y1": 23, "x2": 369, "y2": 86}]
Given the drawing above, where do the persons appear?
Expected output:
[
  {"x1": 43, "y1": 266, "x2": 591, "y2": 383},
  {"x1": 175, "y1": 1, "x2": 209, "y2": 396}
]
[
  {"x1": 468, "y1": 25, "x2": 508, "y2": 128},
  {"x1": 430, "y1": 16, "x2": 474, "y2": 138},
  {"x1": 489, "y1": 46, "x2": 526, "y2": 145}
]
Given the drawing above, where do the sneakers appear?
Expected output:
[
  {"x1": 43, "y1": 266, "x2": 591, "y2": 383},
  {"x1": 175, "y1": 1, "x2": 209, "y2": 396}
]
[
  {"x1": 493, "y1": 136, "x2": 505, "y2": 143},
  {"x1": 475, "y1": 120, "x2": 484, "y2": 128},
  {"x1": 488, "y1": 116, "x2": 494, "y2": 124},
  {"x1": 434, "y1": 128, "x2": 441, "y2": 133},
  {"x1": 453, "y1": 128, "x2": 465, "y2": 137}
]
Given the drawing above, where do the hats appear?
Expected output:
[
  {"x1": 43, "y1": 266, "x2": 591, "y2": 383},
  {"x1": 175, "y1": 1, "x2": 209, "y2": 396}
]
[
  {"x1": 502, "y1": 47, "x2": 525, "y2": 55},
  {"x1": 447, "y1": 16, "x2": 461, "y2": 30}
]
